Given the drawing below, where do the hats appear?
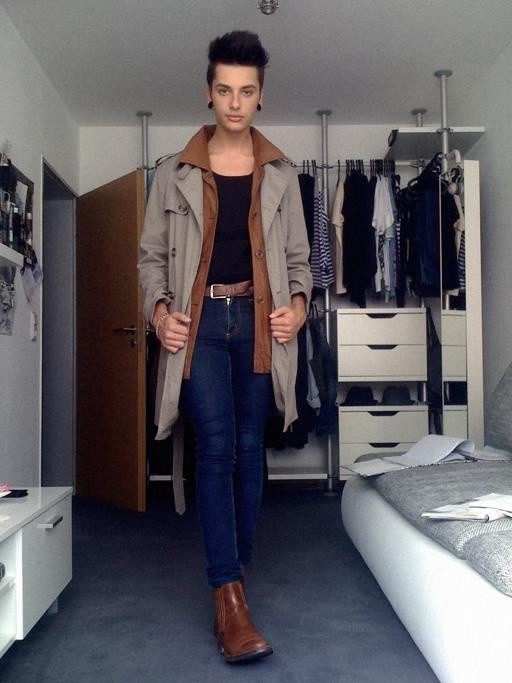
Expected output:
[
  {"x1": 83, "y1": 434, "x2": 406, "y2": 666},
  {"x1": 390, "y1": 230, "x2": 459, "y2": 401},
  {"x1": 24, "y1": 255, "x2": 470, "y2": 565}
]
[{"x1": 340, "y1": 385, "x2": 416, "y2": 406}]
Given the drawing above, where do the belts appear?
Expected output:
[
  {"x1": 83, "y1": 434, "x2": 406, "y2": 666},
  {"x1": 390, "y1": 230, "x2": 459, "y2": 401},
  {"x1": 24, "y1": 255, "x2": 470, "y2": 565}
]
[{"x1": 204, "y1": 280, "x2": 253, "y2": 299}]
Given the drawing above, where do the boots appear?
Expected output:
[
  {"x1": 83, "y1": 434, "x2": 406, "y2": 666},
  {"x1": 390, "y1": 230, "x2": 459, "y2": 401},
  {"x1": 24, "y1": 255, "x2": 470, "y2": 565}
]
[{"x1": 211, "y1": 575, "x2": 274, "y2": 662}]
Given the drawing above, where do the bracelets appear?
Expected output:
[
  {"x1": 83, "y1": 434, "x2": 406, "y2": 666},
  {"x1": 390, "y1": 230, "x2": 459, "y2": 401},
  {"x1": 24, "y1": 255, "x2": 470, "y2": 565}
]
[{"x1": 156, "y1": 312, "x2": 169, "y2": 338}]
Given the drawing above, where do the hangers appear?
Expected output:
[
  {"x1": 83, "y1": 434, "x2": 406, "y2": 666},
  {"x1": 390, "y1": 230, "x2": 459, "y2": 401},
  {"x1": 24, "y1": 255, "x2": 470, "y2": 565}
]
[
  {"x1": 300, "y1": 158, "x2": 399, "y2": 189},
  {"x1": 410, "y1": 150, "x2": 461, "y2": 195}
]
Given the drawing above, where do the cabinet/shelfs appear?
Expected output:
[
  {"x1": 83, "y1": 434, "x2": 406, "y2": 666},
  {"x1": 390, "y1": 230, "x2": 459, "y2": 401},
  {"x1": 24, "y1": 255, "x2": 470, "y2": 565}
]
[
  {"x1": 0, "y1": 486, "x2": 72, "y2": 660},
  {"x1": 326, "y1": 309, "x2": 469, "y2": 485}
]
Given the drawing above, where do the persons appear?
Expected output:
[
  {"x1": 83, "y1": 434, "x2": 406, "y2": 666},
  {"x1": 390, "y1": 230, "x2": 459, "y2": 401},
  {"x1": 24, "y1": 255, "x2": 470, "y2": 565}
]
[{"x1": 136, "y1": 32, "x2": 313, "y2": 664}]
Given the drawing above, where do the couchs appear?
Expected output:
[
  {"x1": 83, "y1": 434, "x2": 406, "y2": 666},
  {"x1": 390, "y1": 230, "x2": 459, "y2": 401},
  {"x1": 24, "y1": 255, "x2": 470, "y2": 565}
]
[{"x1": 339, "y1": 364, "x2": 511, "y2": 682}]
